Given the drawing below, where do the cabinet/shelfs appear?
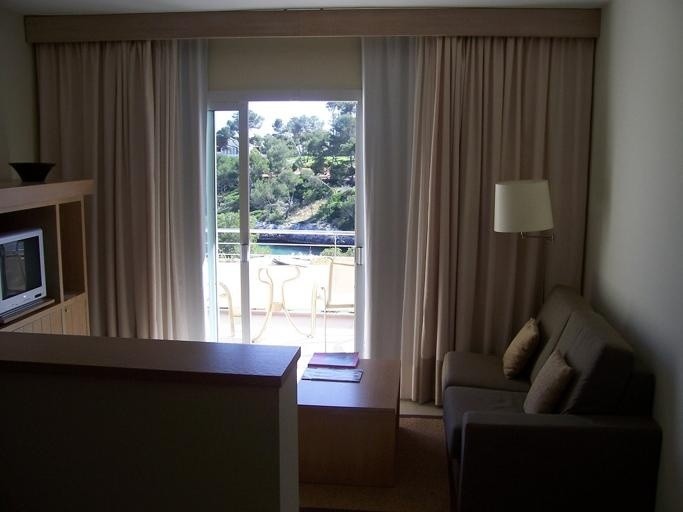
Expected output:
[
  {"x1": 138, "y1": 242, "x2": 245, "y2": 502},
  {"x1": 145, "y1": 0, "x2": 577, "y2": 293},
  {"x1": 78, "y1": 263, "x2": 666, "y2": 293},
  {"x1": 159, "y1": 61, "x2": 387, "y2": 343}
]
[{"x1": 1, "y1": 179, "x2": 97, "y2": 336}]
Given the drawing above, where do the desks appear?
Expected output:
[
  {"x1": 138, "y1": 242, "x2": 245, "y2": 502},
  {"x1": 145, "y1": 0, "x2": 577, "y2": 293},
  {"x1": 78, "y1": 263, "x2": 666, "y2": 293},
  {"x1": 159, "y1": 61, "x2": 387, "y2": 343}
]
[{"x1": 0, "y1": 332, "x2": 300, "y2": 512}]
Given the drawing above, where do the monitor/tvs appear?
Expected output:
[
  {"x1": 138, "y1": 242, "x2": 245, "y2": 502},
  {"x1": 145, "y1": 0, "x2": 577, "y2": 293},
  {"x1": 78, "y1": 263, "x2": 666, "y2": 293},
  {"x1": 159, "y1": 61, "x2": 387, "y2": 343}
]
[{"x1": 0, "y1": 228, "x2": 47, "y2": 318}]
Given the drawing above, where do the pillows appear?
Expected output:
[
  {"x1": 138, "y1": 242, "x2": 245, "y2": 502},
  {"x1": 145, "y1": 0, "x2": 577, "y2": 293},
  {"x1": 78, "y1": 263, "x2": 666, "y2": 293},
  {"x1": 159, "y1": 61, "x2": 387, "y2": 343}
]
[
  {"x1": 502, "y1": 317, "x2": 540, "y2": 380},
  {"x1": 522, "y1": 350, "x2": 576, "y2": 415}
]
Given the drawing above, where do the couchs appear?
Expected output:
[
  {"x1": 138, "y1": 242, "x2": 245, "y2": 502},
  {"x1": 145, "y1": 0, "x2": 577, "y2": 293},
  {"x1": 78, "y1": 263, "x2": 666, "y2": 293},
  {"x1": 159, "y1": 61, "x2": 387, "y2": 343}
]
[{"x1": 441, "y1": 284, "x2": 663, "y2": 508}]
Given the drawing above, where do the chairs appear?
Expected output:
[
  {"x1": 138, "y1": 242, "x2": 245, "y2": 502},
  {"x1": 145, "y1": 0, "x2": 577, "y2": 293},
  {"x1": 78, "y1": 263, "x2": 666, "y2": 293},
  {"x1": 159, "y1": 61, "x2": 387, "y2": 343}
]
[{"x1": 213, "y1": 256, "x2": 355, "y2": 353}]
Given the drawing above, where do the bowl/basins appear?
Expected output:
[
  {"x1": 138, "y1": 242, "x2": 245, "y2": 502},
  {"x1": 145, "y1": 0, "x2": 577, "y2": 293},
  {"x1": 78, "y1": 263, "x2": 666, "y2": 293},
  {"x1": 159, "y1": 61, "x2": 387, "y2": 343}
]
[{"x1": 7, "y1": 162, "x2": 55, "y2": 184}]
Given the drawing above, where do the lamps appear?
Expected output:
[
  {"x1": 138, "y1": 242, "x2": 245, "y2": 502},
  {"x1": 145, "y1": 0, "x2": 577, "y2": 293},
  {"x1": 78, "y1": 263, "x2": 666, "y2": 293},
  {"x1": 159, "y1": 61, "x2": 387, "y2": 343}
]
[{"x1": 494, "y1": 179, "x2": 555, "y2": 243}]
